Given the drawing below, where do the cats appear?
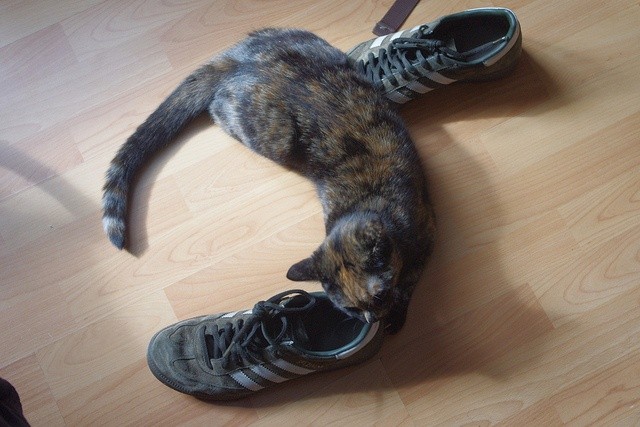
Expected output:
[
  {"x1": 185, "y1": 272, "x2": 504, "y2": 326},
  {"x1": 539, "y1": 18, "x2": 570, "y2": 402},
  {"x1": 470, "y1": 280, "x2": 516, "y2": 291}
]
[{"x1": 98, "y1": 25, "x2": 438, "y2": 327}]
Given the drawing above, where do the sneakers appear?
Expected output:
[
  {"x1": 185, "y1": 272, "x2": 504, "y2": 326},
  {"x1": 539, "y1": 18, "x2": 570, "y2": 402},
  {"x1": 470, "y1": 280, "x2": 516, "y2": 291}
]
[
  {"x1": 145, "y1": 288, "x2": 387, "y2": 403},
  {"x1": 345, "y1": 4, "x2": 523, "y2": 115}
]
